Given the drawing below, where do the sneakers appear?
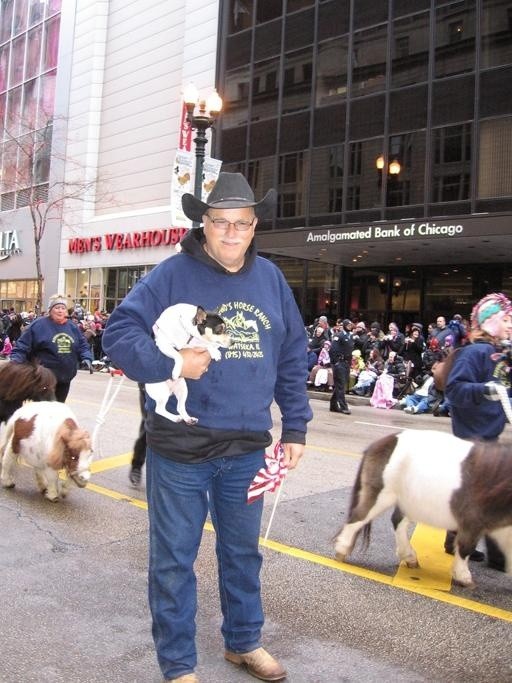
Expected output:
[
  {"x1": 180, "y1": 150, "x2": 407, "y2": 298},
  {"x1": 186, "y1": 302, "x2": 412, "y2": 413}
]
[
  {"x1": 444, "y1": 549, "x2": 485, "y2": 562},
  {"x1": 167, "y1": 672, "x2": 199, "y2": 683},
  {"x1": 130, "y1": 469, "x2": 142, "y2": 484},
  {"x1": 224, "y1": 647, "x2": 287, "y2": 681}
]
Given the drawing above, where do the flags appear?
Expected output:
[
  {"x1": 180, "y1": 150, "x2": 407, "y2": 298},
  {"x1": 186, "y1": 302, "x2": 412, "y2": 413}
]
[{"x1": 247, "y1": 438, "x2": 289, "y2": 507}]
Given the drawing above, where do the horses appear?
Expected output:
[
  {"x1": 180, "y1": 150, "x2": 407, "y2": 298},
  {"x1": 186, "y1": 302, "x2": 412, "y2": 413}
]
[
  {"x1": 0, "y1": 397, "x2": 93, "y2": 504},
  {"x1": 0, "y1": 356, "x2": 57, "y2": 425},
  {"x1": 330, "y1": 428, "x2": 512, "y2": 591}
]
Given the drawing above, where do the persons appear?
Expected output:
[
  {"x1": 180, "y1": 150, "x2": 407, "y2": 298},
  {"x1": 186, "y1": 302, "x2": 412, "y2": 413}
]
[
  {"x1": 127, "y1": 369, "x2": 161, "y2": 488},
  {"x1": 443, "y1": 292, "x2": 511, "y2": 566},
  {"x1": 308, "y1": 292, "x2": 512, "y2": 416},
  {"x1": 102, "y1": 171, "x2": 312, "y2": 683},
  {"x1": 0, "y1": 295, "x2": 131, "y2": 402}
]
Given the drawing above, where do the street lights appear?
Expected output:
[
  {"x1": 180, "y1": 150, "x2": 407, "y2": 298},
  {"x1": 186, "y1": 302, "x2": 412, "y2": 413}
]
[
  {"x1": 181, "y1": 86, "x2": 224, "y2": 234},
  {"x1": 375, "y1": 151, "x2": 401, "y2": 217},
  {"x1": 324, "y1": 299, "x2": 337, "y2": 318},
  {"x1": 378, "y1": 275, "x2": 401, "y2": 332}
]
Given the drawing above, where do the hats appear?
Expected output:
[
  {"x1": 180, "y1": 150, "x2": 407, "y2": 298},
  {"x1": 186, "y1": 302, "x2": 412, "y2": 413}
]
[
  {"x1": 48, "y1": 294, "x2": 68, "y2": 311},
  {"x1": 470, "y1": 292, "x2": 512, "y2": 338},
  {"x1": 181, "y1": 172, "x2": 278, "y2": 223}
]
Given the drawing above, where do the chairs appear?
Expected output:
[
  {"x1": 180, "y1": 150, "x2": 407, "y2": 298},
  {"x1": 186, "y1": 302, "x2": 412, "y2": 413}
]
[{"x1": 394, "y1": 360, "x2": 411, "y2": 399}]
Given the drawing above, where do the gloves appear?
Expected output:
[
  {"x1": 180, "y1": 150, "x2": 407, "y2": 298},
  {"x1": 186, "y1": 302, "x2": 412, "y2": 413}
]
[{"x1": 80, "y1": 359, "x2": 93, "y2": 374}]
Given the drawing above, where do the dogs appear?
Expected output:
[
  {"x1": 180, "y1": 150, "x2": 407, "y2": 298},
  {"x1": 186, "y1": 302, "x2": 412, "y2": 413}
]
[{"x1": 144, "y1": 302, "x2": 235, "y2": 427}]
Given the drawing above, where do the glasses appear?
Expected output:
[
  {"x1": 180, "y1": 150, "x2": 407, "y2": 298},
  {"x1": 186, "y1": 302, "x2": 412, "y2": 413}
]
[{"x1": 206, "y1": 214, "x2": 257, "y2": 232}]
